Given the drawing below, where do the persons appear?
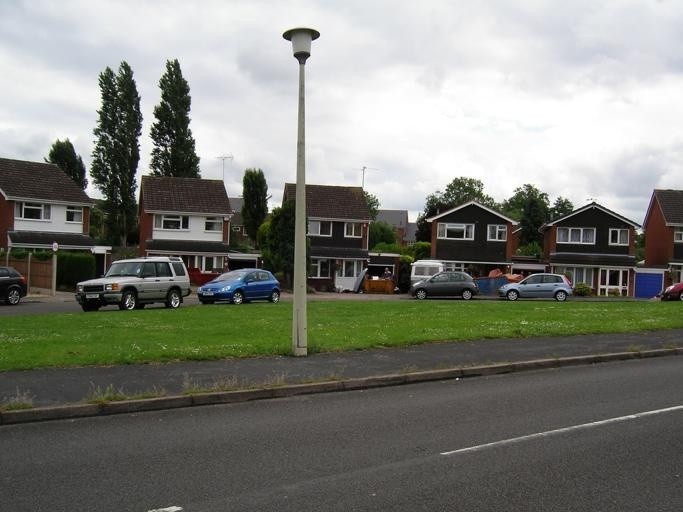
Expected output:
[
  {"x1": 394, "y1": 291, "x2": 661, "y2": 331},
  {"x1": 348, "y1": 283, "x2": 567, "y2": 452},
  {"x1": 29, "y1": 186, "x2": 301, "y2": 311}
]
[{"x1": 382, "y1": 267, "x2": 393, "y2": 280}]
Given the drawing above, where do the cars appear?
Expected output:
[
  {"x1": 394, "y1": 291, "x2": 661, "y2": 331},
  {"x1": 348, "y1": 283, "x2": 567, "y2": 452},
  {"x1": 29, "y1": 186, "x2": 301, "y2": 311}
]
[
  {"x1": 196, "y1": 267, "x2": 281, "y2": 306},
  {"x1": 660, "y1": 282, "x2": 683, "y2": 301},
  {"x1": 496, "y1": 272, "x2": 575, "y2": 302},
  {"x1": 409, "y1": 271, "x2": 480, "y2": 301},
  {"x1": 0, "y1": 266, "x2": 29, "y2": 306}
]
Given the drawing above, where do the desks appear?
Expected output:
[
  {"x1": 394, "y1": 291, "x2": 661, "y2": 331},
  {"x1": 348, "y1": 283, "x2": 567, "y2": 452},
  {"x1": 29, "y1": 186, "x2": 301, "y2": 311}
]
[{"x1": 365, "y1": 279, "x2": 395, "y2": 294}]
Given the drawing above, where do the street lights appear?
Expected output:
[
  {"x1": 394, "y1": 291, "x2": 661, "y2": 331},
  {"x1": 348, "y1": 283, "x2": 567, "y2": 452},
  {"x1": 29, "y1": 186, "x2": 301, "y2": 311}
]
[{"x1": 282, "y1": 26, "x2": 321, "y2": 357}]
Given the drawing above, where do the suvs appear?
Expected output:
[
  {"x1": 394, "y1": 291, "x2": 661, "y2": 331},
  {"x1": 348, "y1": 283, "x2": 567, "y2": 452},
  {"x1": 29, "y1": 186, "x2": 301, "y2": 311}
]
[{"x1": 75, "y1": 256, "x2": 193, "y2": 311}]
